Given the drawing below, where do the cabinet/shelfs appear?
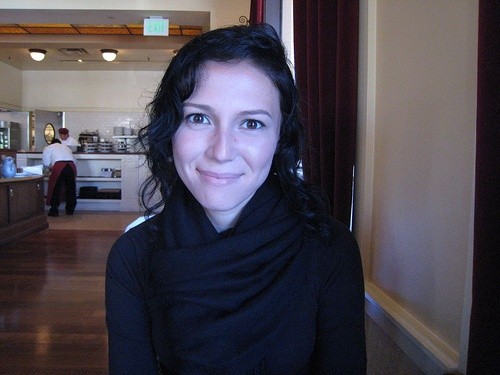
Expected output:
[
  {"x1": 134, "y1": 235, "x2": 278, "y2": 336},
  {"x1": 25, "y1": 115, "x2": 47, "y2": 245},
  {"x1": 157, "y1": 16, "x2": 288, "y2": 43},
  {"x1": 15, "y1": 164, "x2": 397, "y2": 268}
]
[{"x1": 0, "y1": 134, "x2": 165, "y2": 247}]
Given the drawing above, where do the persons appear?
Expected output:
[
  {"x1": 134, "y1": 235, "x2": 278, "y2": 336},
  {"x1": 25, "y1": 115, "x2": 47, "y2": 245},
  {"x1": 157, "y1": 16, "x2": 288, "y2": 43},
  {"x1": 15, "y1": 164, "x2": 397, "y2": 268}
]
[
  {"x1": 42, "y1": 139, "x2": 78, "y2": 217},
  {"x1": 105, "y1": 22, "x2": 368, "y2": 375},
  {"x1": 57, "y1": 127, "x2": 78, "y2": 152}
]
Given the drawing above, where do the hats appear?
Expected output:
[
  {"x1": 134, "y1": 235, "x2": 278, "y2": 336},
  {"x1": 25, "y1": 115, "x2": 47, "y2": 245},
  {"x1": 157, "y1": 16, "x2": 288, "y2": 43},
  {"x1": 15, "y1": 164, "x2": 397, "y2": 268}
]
[{"x1": 58, "y1": 128, "x2": 68, "y2": 135}]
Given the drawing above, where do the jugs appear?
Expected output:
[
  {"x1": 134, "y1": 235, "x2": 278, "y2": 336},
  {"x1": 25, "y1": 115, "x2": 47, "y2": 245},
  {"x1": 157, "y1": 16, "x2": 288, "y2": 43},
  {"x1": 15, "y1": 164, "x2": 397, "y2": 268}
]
[{"x1": 2, "y1": 156, "x2": 16, "y2": 178}]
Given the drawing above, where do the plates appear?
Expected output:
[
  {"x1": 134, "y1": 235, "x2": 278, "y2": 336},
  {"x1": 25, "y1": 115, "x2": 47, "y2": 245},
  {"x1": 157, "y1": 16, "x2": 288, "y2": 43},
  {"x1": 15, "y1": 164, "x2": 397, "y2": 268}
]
[{"x1": 113, "y1": 126, "x2": 139, "y2": 136}]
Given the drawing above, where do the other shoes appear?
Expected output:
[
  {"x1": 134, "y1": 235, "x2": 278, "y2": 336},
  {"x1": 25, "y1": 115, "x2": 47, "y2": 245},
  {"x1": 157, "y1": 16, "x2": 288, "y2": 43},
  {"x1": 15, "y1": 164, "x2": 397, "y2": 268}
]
[
  {"x1": 66, "y1": 209, "x2": 73, "y2": 215},
  {"x1": 48, "y1": 208, "x2": 59, "y2": 216}
]
